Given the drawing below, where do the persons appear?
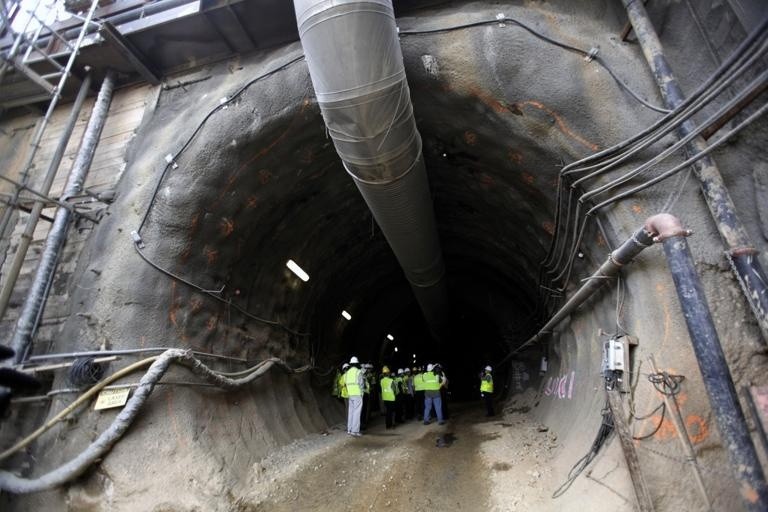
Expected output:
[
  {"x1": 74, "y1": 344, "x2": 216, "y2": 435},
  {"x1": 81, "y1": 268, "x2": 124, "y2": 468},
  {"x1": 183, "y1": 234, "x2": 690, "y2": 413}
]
[
  {"x1": 480, "y1": 365, "x2": 495, "y2": 417},
  {"x1": 338, "y1": 356, "x2": 450, "y2": 437}
]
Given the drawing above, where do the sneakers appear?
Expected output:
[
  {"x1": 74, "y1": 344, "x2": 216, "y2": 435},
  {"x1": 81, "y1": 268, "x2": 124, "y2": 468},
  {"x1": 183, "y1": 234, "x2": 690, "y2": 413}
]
[
  {"x1": 423, "y1": 420, "x2": 445, "y2": 425},
  {"x1": 350, "y1": 431, "x2": 364, "y2": 438},
  {"x1": 385, "y1": 416, "x2": 415, "y2": 430}
]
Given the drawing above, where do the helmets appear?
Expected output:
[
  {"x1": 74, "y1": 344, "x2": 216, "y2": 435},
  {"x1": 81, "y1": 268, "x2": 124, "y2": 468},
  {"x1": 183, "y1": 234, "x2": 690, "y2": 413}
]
[
  {"x1": 382, "y1": 366, "x2": 418, "y2": 374},
  {"x1": 349, "y1": 356, "x2": 361, "y2": 364},
  {"x1": 484, "y1": 365, "x2": 493, "y2": 372},
  {"x1": 342, "y1": 362, "x2": 350, "y2": 371},
  {"x1": 425, "y1": 363, "x2": 434, "y2": 372},
  {"x1": 360, "y1": 362, "x2": 374, "y2": 370}
]
